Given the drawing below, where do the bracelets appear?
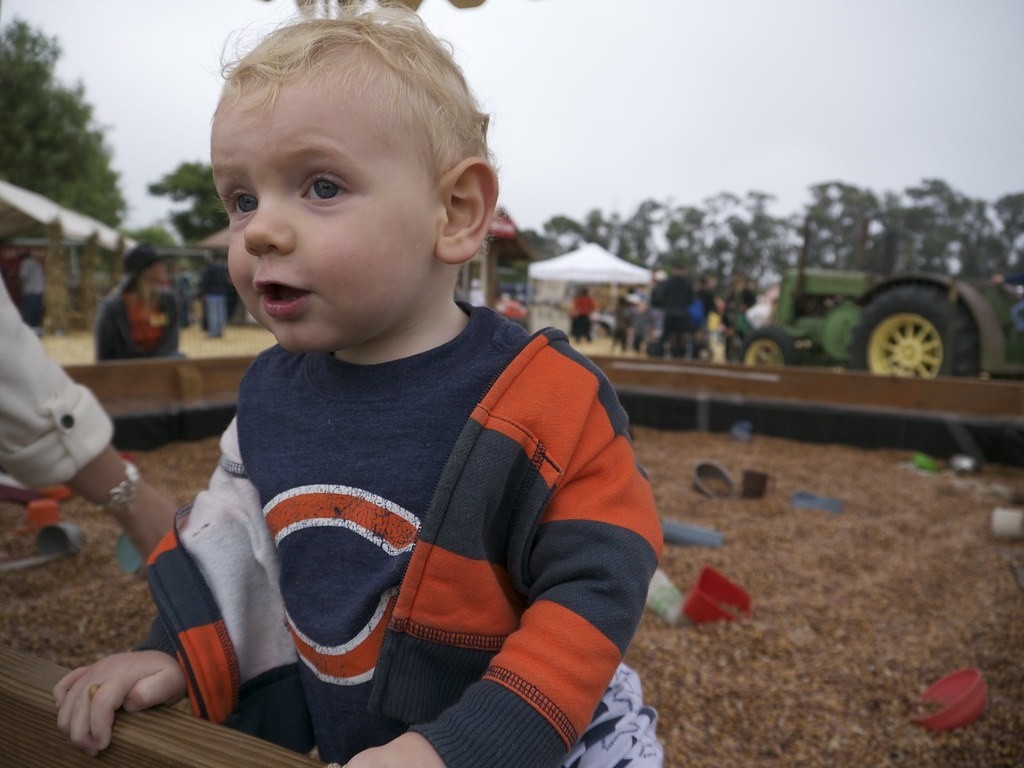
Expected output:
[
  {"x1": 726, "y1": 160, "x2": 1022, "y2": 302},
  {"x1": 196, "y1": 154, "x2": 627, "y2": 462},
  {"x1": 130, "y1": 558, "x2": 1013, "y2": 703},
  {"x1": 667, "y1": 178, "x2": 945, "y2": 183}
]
[{"x1": 100, "y1": 460, "x2": 139, "y2": 512}]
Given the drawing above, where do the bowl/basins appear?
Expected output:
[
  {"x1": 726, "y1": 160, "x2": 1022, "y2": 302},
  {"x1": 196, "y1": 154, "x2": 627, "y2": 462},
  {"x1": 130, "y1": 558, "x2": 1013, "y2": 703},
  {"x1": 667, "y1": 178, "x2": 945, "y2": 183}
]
[
  {"x1": 910, "y1": 667, "x2": 988, "y2": 729},
  {"x1": 694, "y1": 461, "x2": 735, "y2": 499},
  {"x1": 38, "y1": 525, "x2": 85, "y2": 557}
]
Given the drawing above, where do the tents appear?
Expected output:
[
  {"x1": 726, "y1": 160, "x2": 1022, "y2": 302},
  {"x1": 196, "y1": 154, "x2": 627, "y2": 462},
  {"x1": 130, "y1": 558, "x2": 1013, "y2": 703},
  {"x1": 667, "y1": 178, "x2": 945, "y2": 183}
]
[{"x1": 524, "y1": 242, "x2": 655, "y2": 333}]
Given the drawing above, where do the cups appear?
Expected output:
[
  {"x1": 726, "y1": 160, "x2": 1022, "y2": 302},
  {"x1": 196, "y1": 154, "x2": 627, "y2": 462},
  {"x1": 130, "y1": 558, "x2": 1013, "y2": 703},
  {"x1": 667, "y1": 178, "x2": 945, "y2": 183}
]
[{"x1": 743, "y1": 471, "x2": 776, "y2": 498}]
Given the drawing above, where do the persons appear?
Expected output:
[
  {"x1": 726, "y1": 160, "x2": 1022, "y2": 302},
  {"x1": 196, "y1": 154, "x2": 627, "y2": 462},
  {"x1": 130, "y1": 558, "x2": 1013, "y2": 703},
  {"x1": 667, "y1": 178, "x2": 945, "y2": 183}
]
[
  {"x1": 612, "y1": 261, "x2": 755, "y2": 365},
  {"x1": 0, "y1": 277, "x2": 178, "y2": 561},
  {"x1": 993, "y1": 275, "x2": 1024, "y2": 333},
  {"x1": 152, "y1": 253, "x2": 232, "y2": 339},
  {"x1": 54, "y1": 14, "x2": 664, "y2": 768},
  {"x1": 18, "y1": 251, "x2": 45, "y2": 332},
  {"x1": 571, "y1": 284, "x2": 597, "y2": 344},
  {"x1": 96, "y1": 245, "x2": 189, "y2": 360}
]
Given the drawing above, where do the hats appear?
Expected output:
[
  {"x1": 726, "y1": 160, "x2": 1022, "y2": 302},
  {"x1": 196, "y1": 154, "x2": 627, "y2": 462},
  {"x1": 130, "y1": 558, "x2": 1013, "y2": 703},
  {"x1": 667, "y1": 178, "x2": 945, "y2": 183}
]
[{"x1": 122, "y1": 242, "x2": 165, "y2": 275}]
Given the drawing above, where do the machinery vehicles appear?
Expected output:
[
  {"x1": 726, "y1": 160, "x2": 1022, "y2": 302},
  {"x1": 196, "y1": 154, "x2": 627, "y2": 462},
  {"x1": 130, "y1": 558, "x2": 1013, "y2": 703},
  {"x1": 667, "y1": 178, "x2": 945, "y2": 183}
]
[{"x1": 721, "y1": 260, "x2": 1004, "y2": 380}]
